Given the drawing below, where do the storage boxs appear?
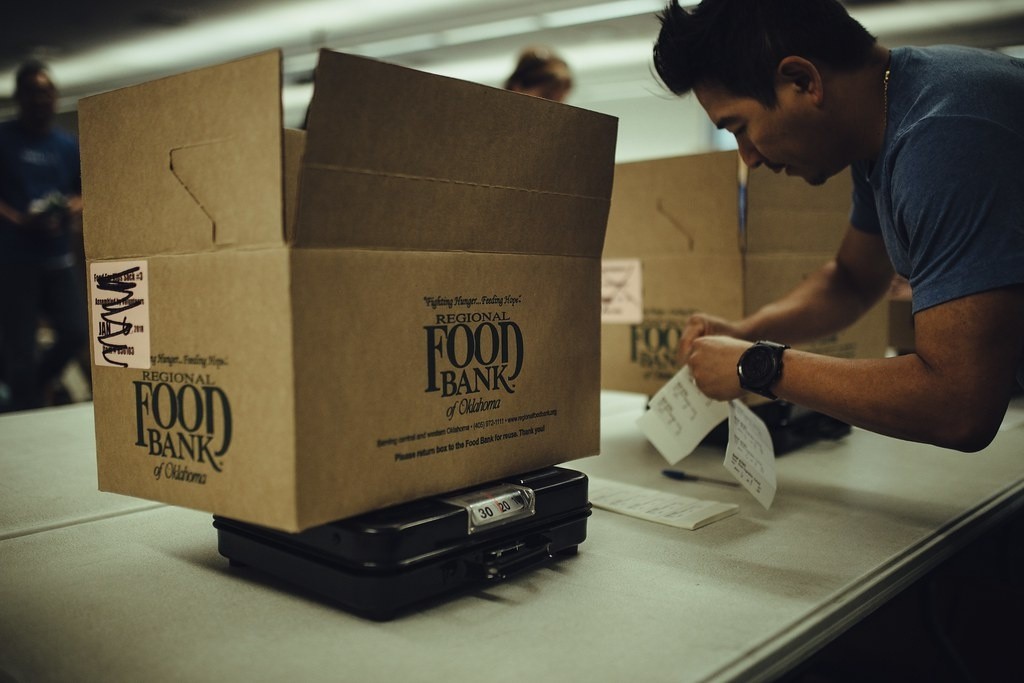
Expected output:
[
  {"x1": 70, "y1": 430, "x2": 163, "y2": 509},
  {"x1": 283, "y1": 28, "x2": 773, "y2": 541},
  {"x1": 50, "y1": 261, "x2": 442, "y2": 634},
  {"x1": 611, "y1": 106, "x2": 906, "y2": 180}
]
[
  {"x1": 78, "y1": 47, "x2": 619, "y2": 534},
  {"x1": 213, "y1": 467, "x2": 592, "y2": 624},
  {"x1": 601, "y1": 150, "x2": 890, "y2": 395}
]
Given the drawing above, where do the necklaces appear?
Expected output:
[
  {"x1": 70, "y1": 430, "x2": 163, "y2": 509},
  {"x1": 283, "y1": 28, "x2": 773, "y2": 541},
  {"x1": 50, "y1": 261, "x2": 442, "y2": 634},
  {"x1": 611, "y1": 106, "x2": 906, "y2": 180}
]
[{"x1": 879, "y1": 49, "x2": 892, "y2": 150}]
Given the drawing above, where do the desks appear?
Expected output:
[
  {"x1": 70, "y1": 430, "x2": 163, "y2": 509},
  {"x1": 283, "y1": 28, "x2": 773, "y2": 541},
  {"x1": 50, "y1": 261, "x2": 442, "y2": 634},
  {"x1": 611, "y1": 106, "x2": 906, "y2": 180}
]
[{"x1": 0, "y1": 396, "x2": 1023, "y2": 683}]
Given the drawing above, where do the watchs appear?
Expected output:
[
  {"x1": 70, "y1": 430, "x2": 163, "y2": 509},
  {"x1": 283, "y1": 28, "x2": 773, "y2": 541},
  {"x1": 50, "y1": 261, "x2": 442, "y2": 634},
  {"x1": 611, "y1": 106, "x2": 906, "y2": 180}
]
[{"x1": 737, "y1": 338, "x2": 793, "y2": 401}]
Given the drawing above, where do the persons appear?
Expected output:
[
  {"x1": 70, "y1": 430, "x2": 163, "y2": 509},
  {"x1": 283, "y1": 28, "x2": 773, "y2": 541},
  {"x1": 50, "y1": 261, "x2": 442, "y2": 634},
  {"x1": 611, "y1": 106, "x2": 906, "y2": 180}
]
[
  {"x1": 653, "y1": 0, "x2": 1024, "y2": 453},
  {"x1": 1, "y1": 60, "x2": 89, "y2": 415},
  {"x1": 504, "y1": 48, "x2": 572, "y2": 102}
]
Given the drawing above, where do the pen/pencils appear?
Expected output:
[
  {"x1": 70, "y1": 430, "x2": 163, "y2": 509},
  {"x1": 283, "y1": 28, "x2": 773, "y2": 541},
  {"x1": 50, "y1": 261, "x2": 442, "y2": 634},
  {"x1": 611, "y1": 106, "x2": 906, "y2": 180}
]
[{"x1": 661, "y1": 468, "x2": 740, "y2": 490}]
